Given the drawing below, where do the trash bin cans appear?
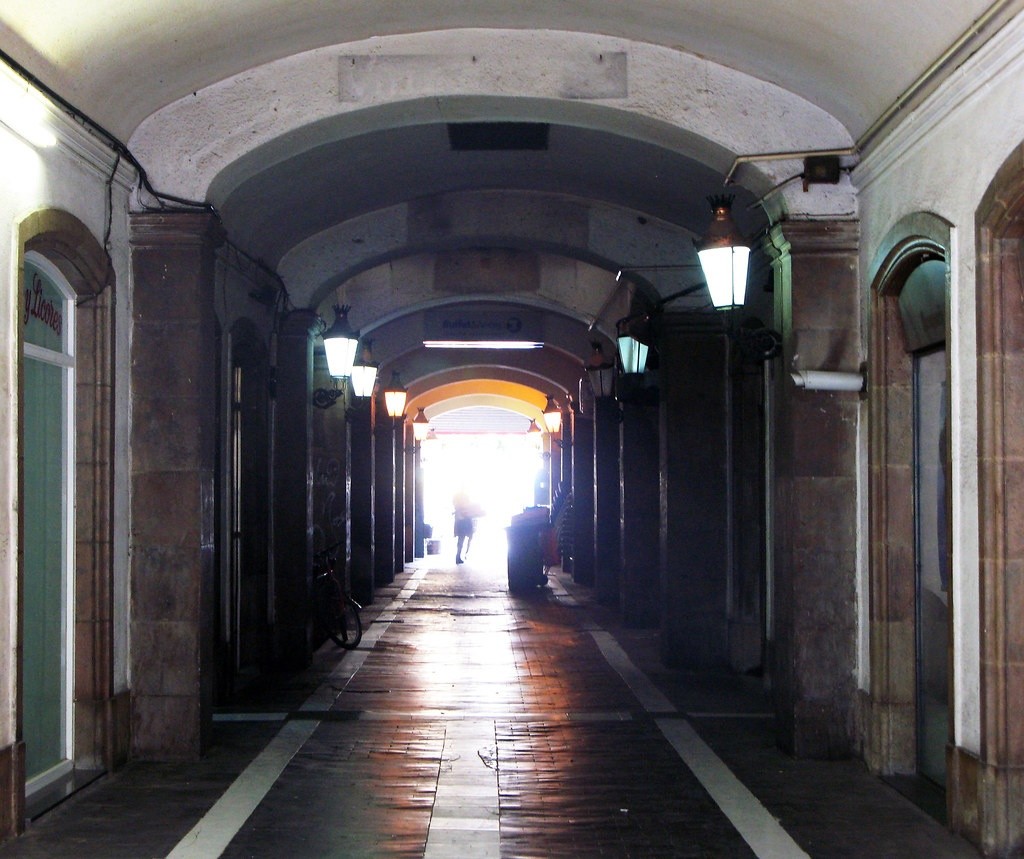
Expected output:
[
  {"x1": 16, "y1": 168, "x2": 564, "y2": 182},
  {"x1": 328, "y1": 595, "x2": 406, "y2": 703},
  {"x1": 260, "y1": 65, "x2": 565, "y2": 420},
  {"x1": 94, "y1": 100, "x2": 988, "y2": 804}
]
[{"x1": 503, "y1": 518, "x2": 550, "y2": 594}]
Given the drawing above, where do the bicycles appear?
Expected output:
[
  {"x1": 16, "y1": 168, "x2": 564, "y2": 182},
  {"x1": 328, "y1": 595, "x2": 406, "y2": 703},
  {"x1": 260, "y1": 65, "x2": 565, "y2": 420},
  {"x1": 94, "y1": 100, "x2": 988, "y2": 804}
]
[{"x1": 310, "y1": 539, "x2": 363, "y2": 650}]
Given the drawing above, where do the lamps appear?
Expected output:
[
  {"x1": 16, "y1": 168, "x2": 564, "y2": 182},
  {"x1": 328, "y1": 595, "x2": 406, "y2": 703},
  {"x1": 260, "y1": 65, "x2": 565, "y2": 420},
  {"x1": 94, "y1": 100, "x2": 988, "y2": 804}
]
[
  {"x1": 526, "y1": 418, "x2": 552, "y2": 461},
  {"x1": 345, "y1": 337, "x2": 380, "y2": 423},
  {"x1": 372, "y1": 368, "x2": 408, "y2": 441},
  {"x1": 313, "y1": 303, "x2": 359, "y2": 409},
  {"x1": 580, "y1": 338, "x2": 621, "y2": 413},
  {"x1": 401, "y1": 406, "x2": 431, "y2": 455},
  {"x1": 541, "y1": 393, "x2": 574, "y2": 449},
  {"x1": 616, "y1": 311, "x2": 653, "y2": 378},
  {"x1": 690, "y1": 192, "x2": 754, "y2": 314}
]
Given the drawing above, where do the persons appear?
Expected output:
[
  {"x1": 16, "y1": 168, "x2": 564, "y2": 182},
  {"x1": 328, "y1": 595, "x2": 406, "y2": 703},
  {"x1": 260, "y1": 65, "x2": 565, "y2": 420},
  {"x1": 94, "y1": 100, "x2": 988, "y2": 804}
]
[{"x1": 454, "y1": 493, "x2": 475, "y2": 564}]
